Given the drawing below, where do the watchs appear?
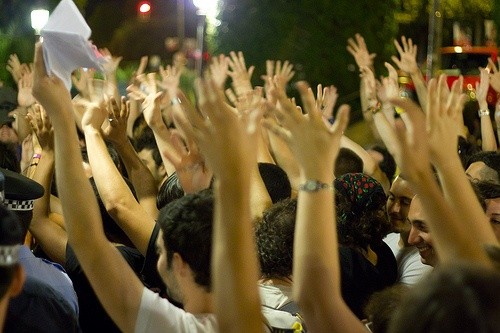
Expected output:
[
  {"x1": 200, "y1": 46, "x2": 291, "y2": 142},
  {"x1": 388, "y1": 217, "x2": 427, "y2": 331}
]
[
  {"x1": 298, "y1": 181, "x2": 333, "y2": 193},
  {"x1": 171, "y1": 98, "x2": 182, "y2": 105}
]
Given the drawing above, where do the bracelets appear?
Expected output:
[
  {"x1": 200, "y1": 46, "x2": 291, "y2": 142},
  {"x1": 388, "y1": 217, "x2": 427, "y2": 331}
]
[
  {"x1": 32, "y1": 154, "x2": 42, "y2": 159},
  {"x1": 31, "y1": 162, "x2": 39, "y2": 166},
  {"x1": 364, "y1": 102, "x2": 381, "y2": 115},
  {"x1": 480, "y1": 109, "x2": 490, "y2": 116}
]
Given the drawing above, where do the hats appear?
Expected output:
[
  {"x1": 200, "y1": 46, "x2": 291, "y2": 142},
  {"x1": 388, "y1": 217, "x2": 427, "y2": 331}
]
[{"x1": 0, "y1": 168, "x2": 45, "y2": 210}]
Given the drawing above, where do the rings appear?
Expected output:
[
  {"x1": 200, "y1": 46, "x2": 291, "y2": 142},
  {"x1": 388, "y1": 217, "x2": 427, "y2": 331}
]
[{"x1": 109, "y1": 119, "x2": 114, "y2": 121}]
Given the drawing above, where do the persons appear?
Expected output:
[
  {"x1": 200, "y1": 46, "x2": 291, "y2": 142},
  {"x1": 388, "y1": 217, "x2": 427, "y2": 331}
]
[{"x1": 0, "y1": 32, "x2": 500, "y2": 333}]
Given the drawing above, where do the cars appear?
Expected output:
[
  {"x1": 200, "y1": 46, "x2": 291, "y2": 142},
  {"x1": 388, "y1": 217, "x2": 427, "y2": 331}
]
[{"x1": 378, "y1": 46, "x2": 500, "y2": 114}]
